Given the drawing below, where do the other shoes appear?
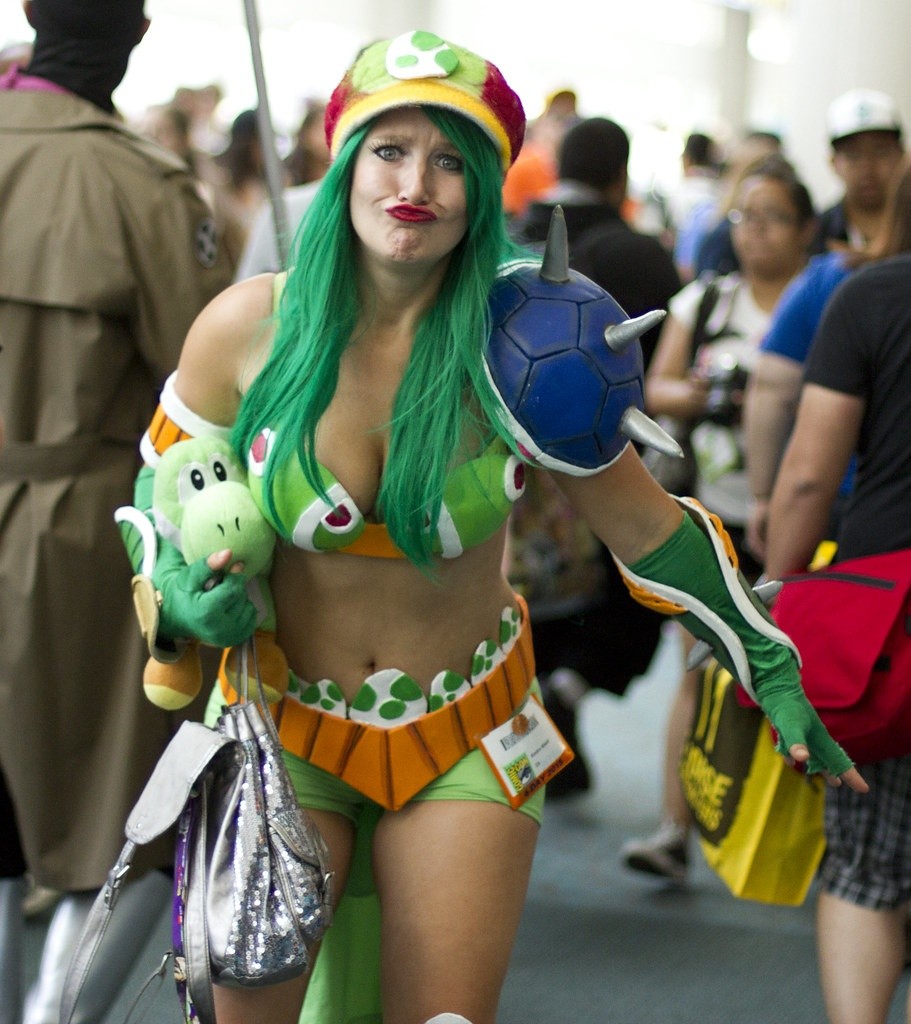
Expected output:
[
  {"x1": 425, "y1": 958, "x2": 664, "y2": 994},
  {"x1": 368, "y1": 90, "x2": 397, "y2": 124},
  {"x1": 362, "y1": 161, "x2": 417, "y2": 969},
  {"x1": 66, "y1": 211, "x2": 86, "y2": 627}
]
[{"x1": 544, "y1": 689, "x2": 589, "y2": 801}]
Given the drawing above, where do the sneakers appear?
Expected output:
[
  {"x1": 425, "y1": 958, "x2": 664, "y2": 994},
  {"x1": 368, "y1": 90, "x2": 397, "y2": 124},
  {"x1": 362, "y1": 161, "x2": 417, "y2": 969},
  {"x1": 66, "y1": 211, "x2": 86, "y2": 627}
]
[{"x1": 628, "y1": 832, "x2": 687, "y2": 878}]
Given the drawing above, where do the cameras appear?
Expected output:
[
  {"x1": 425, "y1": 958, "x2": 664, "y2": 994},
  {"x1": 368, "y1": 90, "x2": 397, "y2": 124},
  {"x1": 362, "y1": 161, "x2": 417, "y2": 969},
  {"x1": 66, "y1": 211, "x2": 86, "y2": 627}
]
[{"x1": 705, "y1": 363, "x2": 750, "y2": 427}]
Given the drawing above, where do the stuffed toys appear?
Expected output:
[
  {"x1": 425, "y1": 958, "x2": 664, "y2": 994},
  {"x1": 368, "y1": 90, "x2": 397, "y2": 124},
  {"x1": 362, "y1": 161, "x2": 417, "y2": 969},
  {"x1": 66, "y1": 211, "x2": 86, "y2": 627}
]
[{"x1": 143, "y1": 435, "x2": 288, "y2": 710}]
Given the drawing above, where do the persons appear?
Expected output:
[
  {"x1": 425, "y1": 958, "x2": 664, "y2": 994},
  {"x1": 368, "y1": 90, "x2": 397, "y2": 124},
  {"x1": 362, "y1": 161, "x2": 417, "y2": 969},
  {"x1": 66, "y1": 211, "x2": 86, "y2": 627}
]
[
  {"x1": 113, "y1": 33, "x2": 872, "y2": 1024},
  {"x1": 0, "y1": 0, "x2": 911, "y2": 1024}
]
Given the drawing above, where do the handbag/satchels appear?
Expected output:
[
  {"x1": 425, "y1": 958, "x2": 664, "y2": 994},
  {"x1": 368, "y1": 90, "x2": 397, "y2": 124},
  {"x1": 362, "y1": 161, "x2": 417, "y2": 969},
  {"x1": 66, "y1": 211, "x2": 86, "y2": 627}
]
[
  {"x1": 640, "y1": 275, "x2": 725, "y2": 497},
  {"x1": 736, "y1": 550, "x2": 911, "y2": 777},
  {"x1": 678, "y1": 656, "x2": 827, "y2": 907}
]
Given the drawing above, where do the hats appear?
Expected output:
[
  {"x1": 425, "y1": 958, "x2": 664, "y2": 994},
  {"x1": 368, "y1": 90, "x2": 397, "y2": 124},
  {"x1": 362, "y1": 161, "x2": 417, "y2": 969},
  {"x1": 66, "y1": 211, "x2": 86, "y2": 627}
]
[
  {"x1": 324, "y1": 30, "x2": 525, "y2": 184},
  {"x1": 546, "y1": 89, "x2": 575, "y2": 111},
  {"x1": 826, "y1": 88, "x2": 900, "y2": 151}
]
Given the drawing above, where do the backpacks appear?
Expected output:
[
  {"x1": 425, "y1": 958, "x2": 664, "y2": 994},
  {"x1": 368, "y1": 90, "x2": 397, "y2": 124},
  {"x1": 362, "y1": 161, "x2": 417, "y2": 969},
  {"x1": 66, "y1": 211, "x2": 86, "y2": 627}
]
[{"x1": 59, "y1": 635, "x2": 335, "y2": 1024}]
